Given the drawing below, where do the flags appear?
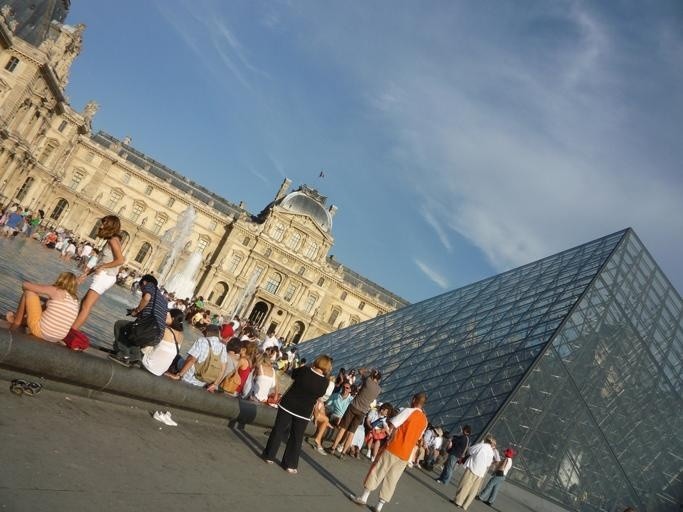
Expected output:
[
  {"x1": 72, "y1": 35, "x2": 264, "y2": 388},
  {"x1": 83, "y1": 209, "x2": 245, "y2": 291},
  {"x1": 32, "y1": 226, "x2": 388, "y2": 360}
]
[{"x1": 319, "y1": 171, "x2": 325, "y2": 178}]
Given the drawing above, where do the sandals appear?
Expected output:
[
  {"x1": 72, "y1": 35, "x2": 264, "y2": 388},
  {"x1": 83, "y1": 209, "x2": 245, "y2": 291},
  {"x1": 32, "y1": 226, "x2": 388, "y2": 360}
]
[
  {"x1": 10, "y1": 378, "x2": 28, "y2": 396},
  {"x1": 24, "y1": 382, "x2": 42, "y2": 397}
]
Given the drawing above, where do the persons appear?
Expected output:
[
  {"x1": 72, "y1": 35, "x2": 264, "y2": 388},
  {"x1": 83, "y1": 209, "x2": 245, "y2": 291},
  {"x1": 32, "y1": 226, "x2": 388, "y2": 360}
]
[
  {"x1": 347, "y1": 391, "x2": 429, "y2": 512},
  {"x1": 474, "y1": 448, "x2": 514, "y2": 507},
  {"x1": 490, "y1": 436, "x2": 502, "y2": 466},
  {"x1": 435, "y1": 423, "x2": 472, "y2": 486},
  {"x1": 449, "y1": 432, "x2": 496, "y2": 512},
  {"x1": 0, "y1": 200, "x2": 452, "y2": 476}
]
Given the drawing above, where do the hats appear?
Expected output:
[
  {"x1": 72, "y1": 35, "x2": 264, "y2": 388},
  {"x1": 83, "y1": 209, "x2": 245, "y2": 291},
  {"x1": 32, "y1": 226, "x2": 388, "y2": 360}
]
[
  {"x1": 434, "y1": 428, "x2": 443, "y2": 437},
  {"x1": 503, "y1": 449, "x2": 512, "y2": 458}
]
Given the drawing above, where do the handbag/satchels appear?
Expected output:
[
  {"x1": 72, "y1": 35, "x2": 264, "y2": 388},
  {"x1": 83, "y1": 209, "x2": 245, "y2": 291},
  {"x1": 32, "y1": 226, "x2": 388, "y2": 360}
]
[
  {"x1": 373, "y1": 429, "x2": 386, "y2": 440},
  {"x1": 168, "y1": 354, "x2": 185, "y2": 375},
  {"x1": 219, "y1": 368, "x2": 242, "y2": 394},
  {"x1": 64, "y1": 328, "x2": 89, "y2": 352},
  {"x1": 124, "y1": 314, "x2": 162, "y2": 346},
  {"x1": 495, "y1": 470, "x2": 502, "y2": 476},
  {"x1": 239, "y1": 368, "x2": 254, "y2": 400}
]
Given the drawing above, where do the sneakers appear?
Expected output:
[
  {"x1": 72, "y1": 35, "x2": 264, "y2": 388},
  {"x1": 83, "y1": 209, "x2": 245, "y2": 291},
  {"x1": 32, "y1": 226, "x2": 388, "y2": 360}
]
[
  {"x1": 324, "y1": 447, "x2": 334, "y2": 455},
  {"x1": 152, "y1": 410, "x2": 173, "y2": 426},
  {"x1": 314, "y1": 447, "x2": 327, "y2": 456},
  {"x1": 108, "y1": 354, "x2": 131, "y2": 368},
  {"x1": 349, "y1": 494, "x2": 366, "y2": 505},
  {"x1": 160, "y1": 410, "x2": 177, "y2": 427},
  {"x1": 365, "y1": 450, "x2": 371, "y2": 459},
  {"x1": 131, "y1": 360, "x2": 141, "y2": 369}
]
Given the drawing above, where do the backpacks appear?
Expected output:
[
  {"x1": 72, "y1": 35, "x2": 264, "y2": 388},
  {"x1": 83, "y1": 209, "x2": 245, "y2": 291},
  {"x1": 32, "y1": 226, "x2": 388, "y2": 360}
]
[{"x1": 194, "y1": 339, "x2": 223, "y2": 385}]
